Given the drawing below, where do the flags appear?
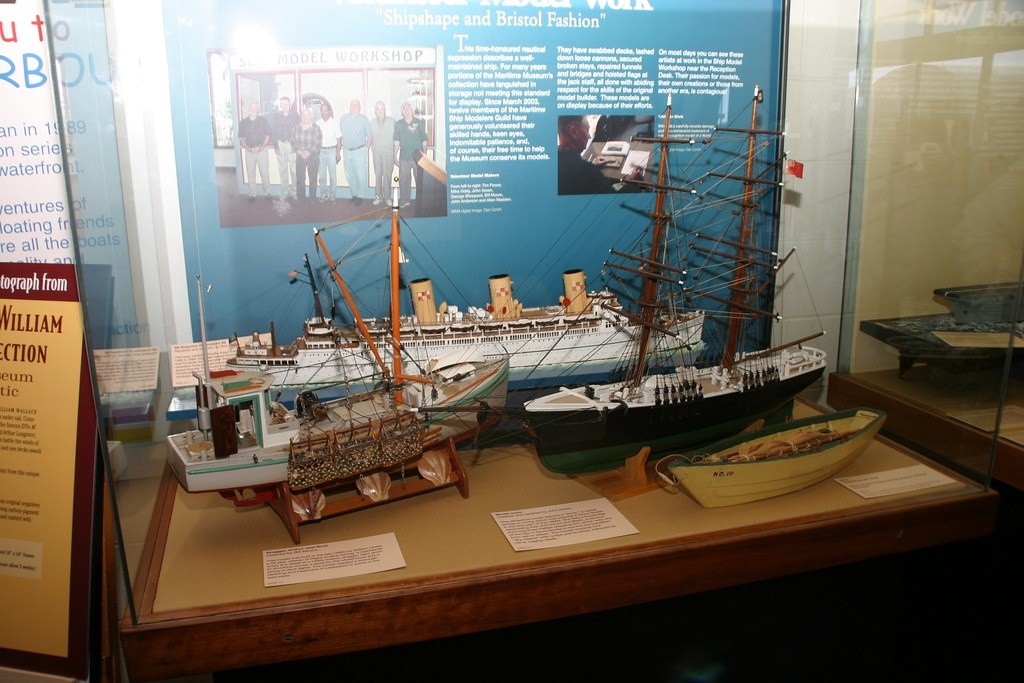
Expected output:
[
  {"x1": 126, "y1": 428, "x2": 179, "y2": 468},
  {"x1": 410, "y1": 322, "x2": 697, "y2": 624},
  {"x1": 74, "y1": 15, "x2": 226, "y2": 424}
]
[{"x1": 785, "y1": 159, "x2": 804, "y2": 179}]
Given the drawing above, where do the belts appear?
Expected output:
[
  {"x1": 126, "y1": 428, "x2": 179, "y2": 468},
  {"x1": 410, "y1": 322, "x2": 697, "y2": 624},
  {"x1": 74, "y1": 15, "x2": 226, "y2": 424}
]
[
  {"x1": 282, "y1": 139, "x2": 290, "y2": 143},
  {"x1": 322, "y1": 145, "x2": 336, "y2": 149},
  {"x1": 349, "y1": 145, "x2": 364, "y2": 150}
]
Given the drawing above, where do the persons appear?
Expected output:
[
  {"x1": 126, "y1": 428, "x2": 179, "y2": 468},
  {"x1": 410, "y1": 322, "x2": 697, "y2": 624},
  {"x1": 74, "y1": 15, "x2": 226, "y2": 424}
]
[
  {"x1": 558, "y1": 115, "x2": 643, "y2": 195},
  {"x1": 235, "y1": 95, "x2": 428, "y2": 208}
]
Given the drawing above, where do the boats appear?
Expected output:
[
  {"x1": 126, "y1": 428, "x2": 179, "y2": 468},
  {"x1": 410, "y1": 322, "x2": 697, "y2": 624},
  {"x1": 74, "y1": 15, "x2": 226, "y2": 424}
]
[{"x1": 667, "y1": 405, "x2": 888, "y2": 509}]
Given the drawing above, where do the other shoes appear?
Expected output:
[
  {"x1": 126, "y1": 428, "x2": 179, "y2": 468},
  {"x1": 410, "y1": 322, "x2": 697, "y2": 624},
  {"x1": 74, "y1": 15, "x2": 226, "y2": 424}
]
[
  {"x1": 351, "y1": 196, "x2": 362, "y2": 205},
  {"x1": 265, "y1": 194, "x2": 271, "y2": 200},
  {"x1": 385, "y1": 199, "x2": 393, "y2": 206},
  {"x1": 331, "y1": 197, "x2": 335, "y2": 203},
  {"x1": 399, "y1": 200, "x2": 410, "y2": 207},
  {"x1": 248, "y1": 195, "x2": 255, "y2": 203},
  {"x1": 320, "y1": 195, "x2": 328, "y2": 202},
  {"x1": 373, "y1": 199, "x2": 383, "y2": 204}
]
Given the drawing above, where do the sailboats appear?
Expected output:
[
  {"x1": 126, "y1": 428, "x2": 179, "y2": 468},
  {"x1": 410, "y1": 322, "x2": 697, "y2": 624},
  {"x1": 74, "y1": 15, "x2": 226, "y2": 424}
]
[
  {"x1": 164, "y1": 189, "x2": 509, "y2": 493},
  {"x1": 407, "y1": 85, "x2": 829, "y2": 474}
]
